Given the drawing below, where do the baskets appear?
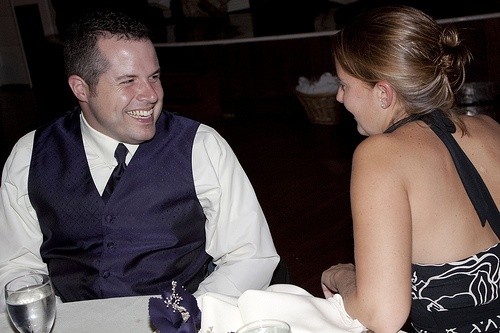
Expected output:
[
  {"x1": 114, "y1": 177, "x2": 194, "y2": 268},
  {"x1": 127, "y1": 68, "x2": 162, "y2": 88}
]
[{"x1": 296, "y1": 87, "x2": 350, "y2": 124}]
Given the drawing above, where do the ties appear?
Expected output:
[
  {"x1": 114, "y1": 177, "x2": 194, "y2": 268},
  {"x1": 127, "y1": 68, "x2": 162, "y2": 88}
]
[{"x1": 101, "y1": 142, "x2": 129, "y2": 204}]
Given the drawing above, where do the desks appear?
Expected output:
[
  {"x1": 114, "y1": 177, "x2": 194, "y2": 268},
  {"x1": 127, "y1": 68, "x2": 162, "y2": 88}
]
[{"x1": 0, "y1": 295, "x2": 156, "y2": 333}]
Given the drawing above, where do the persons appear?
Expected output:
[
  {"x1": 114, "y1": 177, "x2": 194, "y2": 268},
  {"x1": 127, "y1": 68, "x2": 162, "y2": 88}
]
[
  {"x1": 320, "y1": 7, "x2": 500, "y2": 333},
  {"x1": 0, "y1": 12, "x2": 281, "y2": 312}
]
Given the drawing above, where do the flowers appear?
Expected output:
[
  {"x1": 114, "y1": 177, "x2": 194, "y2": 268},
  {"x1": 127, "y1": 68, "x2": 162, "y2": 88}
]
[{"x1": 147, "y1": 281, "x2": 201, "y2": 333}]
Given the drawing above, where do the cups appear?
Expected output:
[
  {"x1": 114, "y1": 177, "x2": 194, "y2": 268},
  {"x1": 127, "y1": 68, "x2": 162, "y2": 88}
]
[
  {"x1": 5, "y1": 274, "x2": 56, "y2": 333},
  {"x1": 236, "y1": 319, "x2": 291, "y2": 333}
]
